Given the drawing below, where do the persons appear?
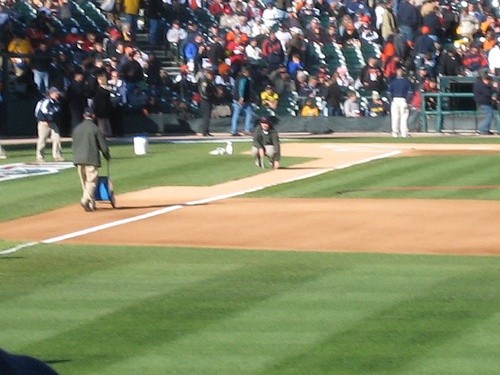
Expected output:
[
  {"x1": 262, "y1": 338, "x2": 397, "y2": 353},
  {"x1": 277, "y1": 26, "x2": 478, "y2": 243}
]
[
  {"x1": 72, "y1": 109, "x2": 112, "y2": 212},
  {"x1": 252, "y1": 117, "x2": 280, "y2": 169},
  {"x1": 0, "y1": 0, "x2": 500, "y2": 137},
  {"x1": 36, "y1": 87, "x2": 65, "y2": 162}
]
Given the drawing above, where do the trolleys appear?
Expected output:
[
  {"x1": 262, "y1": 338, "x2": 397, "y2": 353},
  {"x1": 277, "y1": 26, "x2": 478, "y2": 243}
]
[{"x1": 93, "y1": 159, "x2": 116, "y2": 208}]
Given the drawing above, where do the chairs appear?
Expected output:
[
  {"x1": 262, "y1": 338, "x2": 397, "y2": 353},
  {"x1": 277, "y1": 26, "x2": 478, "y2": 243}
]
[{"x1": 13, "y1": 0, "x2": 500, "y2": 116}]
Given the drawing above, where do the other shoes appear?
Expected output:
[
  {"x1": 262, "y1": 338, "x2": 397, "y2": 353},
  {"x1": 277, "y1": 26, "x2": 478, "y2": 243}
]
[
  {"x1": 55, "y1": 157, "x2": 66, "y2": 162},
  {"x1": 232, "y1": 133, "x2": 242, "y2": 136},
  {"x1": 80, "y1": 201, "x2": 93, "y2": 212},
  {"x1": 480, "y1": 130, "x2": 494, "y2": 136},
  {"x1": 392, "y1": 133, "x2": 399, "y2": 138},
  {"x1": 404, "y1": 134, "x2": 412, "y2": 138},
  {"x1": 203, "y1": 134, "x2": 214, "y2": 138},
  {"x1": 37, "y1": 159, "x2": 46, "y2": 163},
  {"x1": 244, "y1": 132, "x2": 254, "y2": 136}
]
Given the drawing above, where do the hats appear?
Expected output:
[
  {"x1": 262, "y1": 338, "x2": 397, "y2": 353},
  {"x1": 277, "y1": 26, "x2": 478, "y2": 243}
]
[{"x1": 84, "y1": 107, "x2": 94, "y2": 115}]
